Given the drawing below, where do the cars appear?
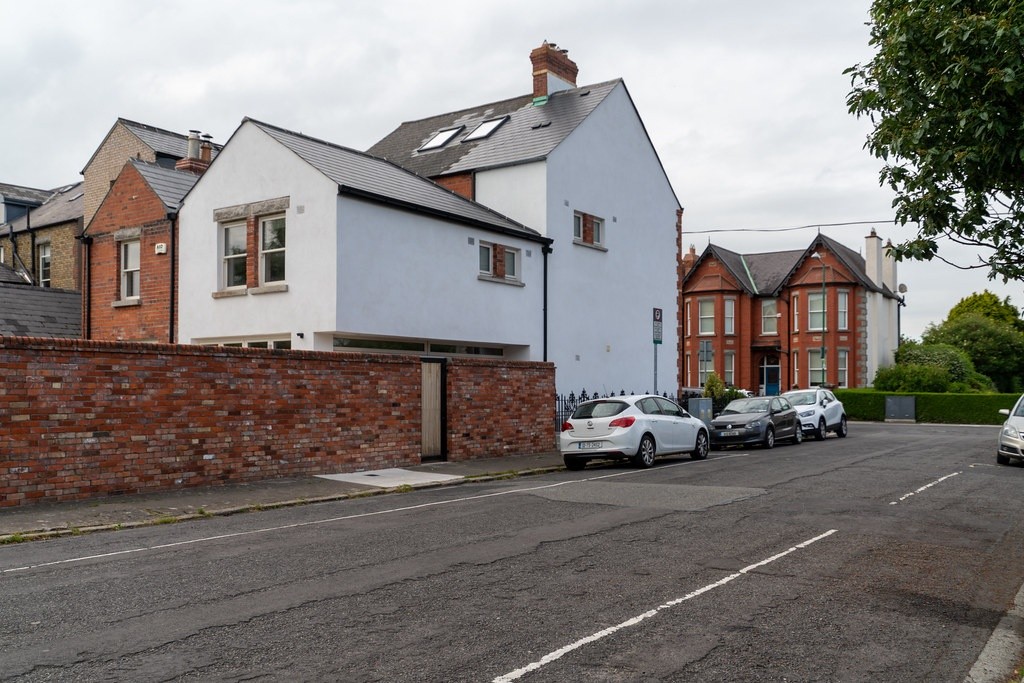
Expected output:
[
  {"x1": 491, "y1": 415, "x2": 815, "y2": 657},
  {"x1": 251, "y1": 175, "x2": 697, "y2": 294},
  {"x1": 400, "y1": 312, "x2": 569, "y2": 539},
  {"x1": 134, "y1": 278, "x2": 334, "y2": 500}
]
[
  {"x1": 997, "y1": 394, "x2": 1024, "y2": 466},
  {"x1": 708, "y1": 395, "x2": 803, "y2": 451},
  {"x1": 560, "y1": 394, "x2": 710, "y2": 471}
]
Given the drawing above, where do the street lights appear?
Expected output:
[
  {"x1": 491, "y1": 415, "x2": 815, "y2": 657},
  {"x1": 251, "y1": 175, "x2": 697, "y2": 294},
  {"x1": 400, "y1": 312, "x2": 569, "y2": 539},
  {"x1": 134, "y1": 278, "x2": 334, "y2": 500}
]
[{"x1": 811, "y1": 251, "x2": 825, "y2": 388}]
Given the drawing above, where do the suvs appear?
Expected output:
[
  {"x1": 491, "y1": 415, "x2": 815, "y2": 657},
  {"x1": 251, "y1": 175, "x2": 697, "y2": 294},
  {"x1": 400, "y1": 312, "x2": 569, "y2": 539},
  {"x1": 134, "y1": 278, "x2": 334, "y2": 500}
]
[{"x1": 779, "y1": 387, "x2": 848, "y2": 441}]
